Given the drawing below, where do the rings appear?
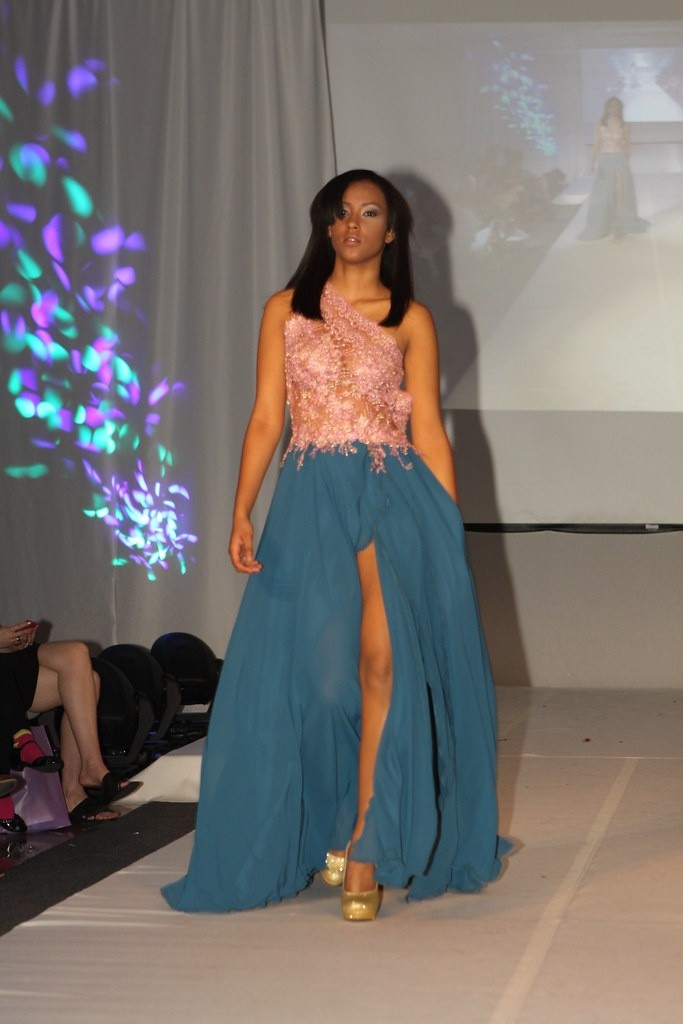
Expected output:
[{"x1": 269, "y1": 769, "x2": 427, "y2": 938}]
[
  {"x1": 17, "y1": 636, "x2": 21, "y2": 640},
  {"x1": 25, "y1": 643, "x2": 32, "y2": 647}
]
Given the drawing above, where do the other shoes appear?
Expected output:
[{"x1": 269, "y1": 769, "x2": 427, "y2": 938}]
[
  {"x1": 9, "y1": 741, "x2": 65, "y2": 773},
  {"x1": 317, "y1": 828, "x2": 381, "y2": 922},
  {"x1": 1, "y1": 813, "x2": 27, "y2": 835}
]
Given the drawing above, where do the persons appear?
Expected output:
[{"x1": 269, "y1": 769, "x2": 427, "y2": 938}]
[
  {"x1": 0, "y1": 620, "x2": 142, "y2": 820},
  {"x1": 231, "y1": 169, "x2": 460, "y2": 923},
  {"x1": 0, "y1": 656, "x2": 62, "y2": 835},
  {"x1": 590, "y1": 97, "x2": 639, "y2": 243}
]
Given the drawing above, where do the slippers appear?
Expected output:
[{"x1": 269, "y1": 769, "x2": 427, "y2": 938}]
[
  {"x1": 84, "y1": 773, "x2": 140, "y2": 803},
  {"x1": 68, "y1": 796, "x2": 120, "y2": 823}
]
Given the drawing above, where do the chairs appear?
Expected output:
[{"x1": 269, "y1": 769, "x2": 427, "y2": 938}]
[{"x1": 36, "y1": 630, "x2": 225, "y2": 780}]
